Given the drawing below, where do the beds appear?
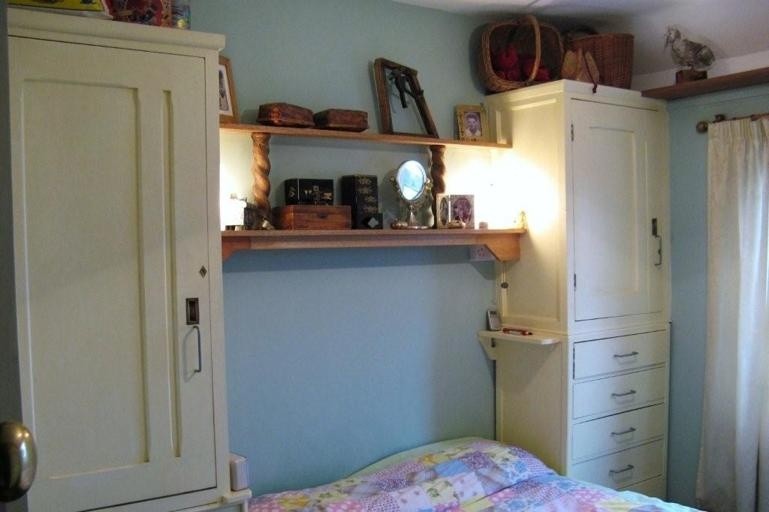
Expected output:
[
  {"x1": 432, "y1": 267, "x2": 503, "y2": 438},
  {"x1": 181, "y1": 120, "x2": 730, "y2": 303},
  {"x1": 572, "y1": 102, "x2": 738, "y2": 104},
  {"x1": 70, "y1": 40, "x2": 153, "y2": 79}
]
[{"x1": 249, "y1": 436, "x2": 708, "y2": 512}]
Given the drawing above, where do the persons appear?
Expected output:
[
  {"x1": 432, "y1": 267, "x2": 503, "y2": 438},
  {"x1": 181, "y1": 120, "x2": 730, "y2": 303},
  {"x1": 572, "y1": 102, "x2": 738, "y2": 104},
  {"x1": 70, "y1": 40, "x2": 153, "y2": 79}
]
[{"x1": 463, "y1": 111, "x2": 482, "y2": 137}]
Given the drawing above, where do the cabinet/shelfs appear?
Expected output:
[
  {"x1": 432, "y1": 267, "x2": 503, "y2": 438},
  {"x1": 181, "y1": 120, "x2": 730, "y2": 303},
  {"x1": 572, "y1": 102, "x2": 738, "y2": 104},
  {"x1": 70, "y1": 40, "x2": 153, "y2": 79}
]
[{"x1": 0, "y1": 4, "x2": 252, "y2": 512}]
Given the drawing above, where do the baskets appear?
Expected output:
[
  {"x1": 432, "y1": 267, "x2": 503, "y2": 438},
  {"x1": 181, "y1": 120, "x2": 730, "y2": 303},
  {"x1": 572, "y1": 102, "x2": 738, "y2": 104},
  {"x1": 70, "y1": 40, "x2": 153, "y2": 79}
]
[
  {"x1": 569, "y1": 31, "x2": 634, "y2": 88},
  {"x1": 475, "y1": 12, "x2": 564, "y2": 93}
]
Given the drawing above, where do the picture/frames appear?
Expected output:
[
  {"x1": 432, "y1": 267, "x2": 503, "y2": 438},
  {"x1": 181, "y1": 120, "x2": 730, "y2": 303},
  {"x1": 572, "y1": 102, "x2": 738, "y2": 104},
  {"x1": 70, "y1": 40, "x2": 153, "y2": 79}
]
[
  {"x1": 456, "y1": 103, "x2": 490, "y2": 143},
  {"x1": 435, "y1": 193, "x2": 475, "y2": 228},
  {"x1": 219, "y1": 54, "x2": 240, "y2": 124}
]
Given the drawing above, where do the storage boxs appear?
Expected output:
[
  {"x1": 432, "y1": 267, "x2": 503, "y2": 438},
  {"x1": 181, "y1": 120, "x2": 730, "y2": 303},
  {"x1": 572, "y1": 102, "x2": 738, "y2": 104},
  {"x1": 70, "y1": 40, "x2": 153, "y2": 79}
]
[{"x1": 270, "y1": 205, "x2": 352, "y2": 230}]
